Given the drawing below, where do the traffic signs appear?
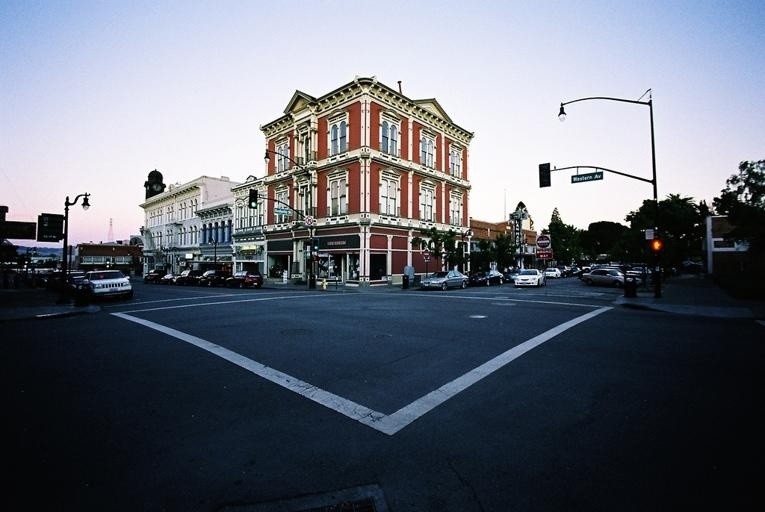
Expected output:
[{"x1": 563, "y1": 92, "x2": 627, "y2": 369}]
[
  {"x1": 536, "y1": 249, "x2": 554, "y2": 260},
  {"x1": 572, "y1": 172, "x2": 603, "y2": 183}
]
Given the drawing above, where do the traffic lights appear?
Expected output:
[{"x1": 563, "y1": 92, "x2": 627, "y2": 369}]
[
  {"x1": 249, "y1": 190, "x2": 258, "y2": 209},
  {"x1": 539, "y1": 163, "x2": 551, "y2": 188},
  {"x1": 653, "y1": 240, "x2": 662, "y2": 251}
]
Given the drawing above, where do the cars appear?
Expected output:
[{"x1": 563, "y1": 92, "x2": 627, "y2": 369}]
[
  {"x1": 503, "y1": 264, "x2": 676, "y2": 288},
  {"x1": 471, "y1": 272, "x2": 505, "y2": 287},
  {"x1": 144, "y1": 269, "x2": 264, "y2": 289},
  {"x1": 67, "y1": 270, "x2": 134, "y2": 302},
  {"x1": 419, "y1": 271, "x2": 470, "y2": 290}
]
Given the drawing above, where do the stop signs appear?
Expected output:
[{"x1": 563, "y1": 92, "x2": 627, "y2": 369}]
[{"x1": 535, "y1": 235, "x2": 551, "y2": 250}]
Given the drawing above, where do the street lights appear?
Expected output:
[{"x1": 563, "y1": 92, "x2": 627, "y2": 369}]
[
  {"x1": 265, "y1": 149, "x2": 316, "y2": 289},
  {"x1": 63, "y1": 193, "x2": 91, "y2": 273},
  {"x1": 557, "y1": 97, "x2": 658, "y2": 203}
]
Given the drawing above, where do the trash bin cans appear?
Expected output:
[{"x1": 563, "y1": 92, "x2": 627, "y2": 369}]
[
  {"x1": 624, "y1": 275, "x2": 636, "y2": 297},
  {"x1": 309, "y1": 275, "x2": 316, "y2": 289},
  {"x1": 403, "y1": 275, "x2": 409, "y2": 289}
]
